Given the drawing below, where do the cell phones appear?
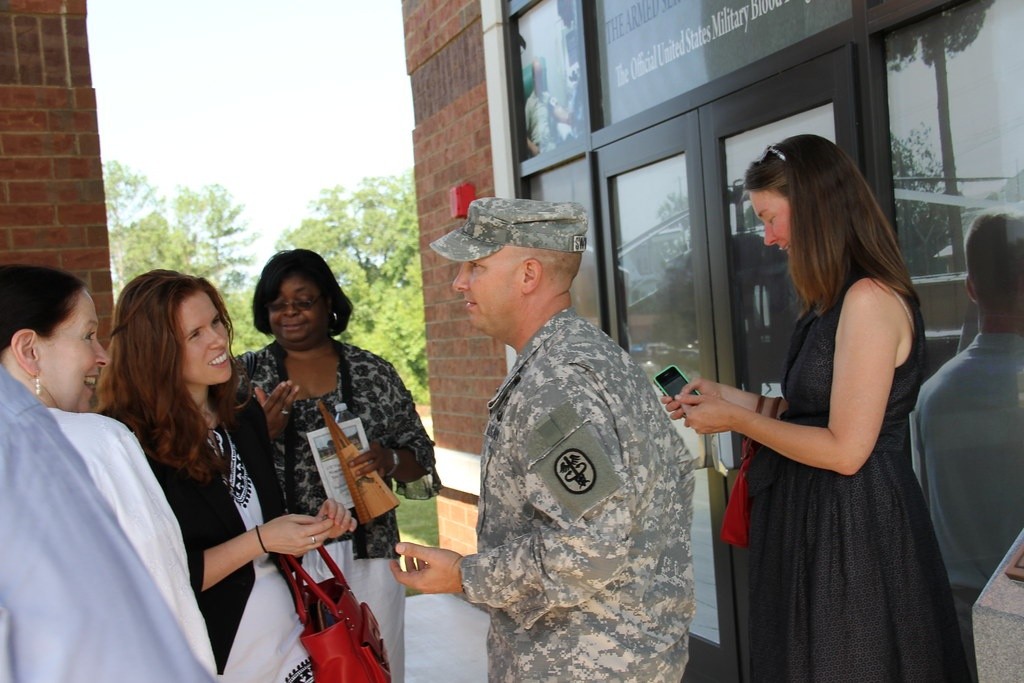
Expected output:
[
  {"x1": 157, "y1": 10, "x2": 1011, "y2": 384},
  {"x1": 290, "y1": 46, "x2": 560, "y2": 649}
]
[{"x1": 653, "y1": 364, "x2": 701, "y2": 418}]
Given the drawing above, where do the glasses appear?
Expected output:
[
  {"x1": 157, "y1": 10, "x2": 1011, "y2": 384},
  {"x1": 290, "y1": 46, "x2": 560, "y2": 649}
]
[
  {"x1": 758, "y1": 143, "x2": 786, "y2": 163},
  {"x1": 266, "y1": 295, "x2": 327, "y2": 313}
]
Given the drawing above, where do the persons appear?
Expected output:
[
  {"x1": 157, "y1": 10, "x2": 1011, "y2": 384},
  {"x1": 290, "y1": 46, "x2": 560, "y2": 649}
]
[
  {"x1": 912, "y1": 213, "x2": 1024, "y2": 671},
  {"x1": 0, "y1": 364, "x2": 216, "y2": 683},
  {"x1": 524, "y1": 58, "x2": 585, "y2": 156},
  {"x1": 389, "y1": 197, "x2": 697, "y2": 682},
  {"x1": 94, "y1": 269, "x2": 358, "y2": 683},
  {"x1": 228, "y1": 249, "x2": 444, "y2": 682},
  {"x1": 0, "y1": 263, "x2": 219, "y2": 683},
  {"x1": 659, "y1": 135, "x2": 977, "y2": 683}
]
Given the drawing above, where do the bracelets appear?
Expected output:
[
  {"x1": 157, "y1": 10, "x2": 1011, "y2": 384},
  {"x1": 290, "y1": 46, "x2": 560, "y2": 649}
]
[{"x1": 256, "y1": 525, "x2": 269, "y2": 554}]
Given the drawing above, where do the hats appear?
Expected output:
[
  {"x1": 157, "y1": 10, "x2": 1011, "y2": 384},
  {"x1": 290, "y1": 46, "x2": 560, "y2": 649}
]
[{"x1": 429, "y1": 197, "x2": 589, "y2": 262}]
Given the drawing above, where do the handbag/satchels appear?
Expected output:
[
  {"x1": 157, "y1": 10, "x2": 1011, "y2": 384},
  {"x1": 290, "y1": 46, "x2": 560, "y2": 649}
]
[
  {"x1": 278, "y1": 542, "x2": 392, "y2": 683},
  {"x1": 720, "y1": 396, "x2": 783, "y2": 548}
]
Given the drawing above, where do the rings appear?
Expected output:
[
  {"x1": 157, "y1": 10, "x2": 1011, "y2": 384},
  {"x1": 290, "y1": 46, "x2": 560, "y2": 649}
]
[
  {"x1": 312, "y1": 536, "x2": 316, "y2": 544},
  {"x1": 282, "y1": 408, "x2": 288, "y2": 414}
]
